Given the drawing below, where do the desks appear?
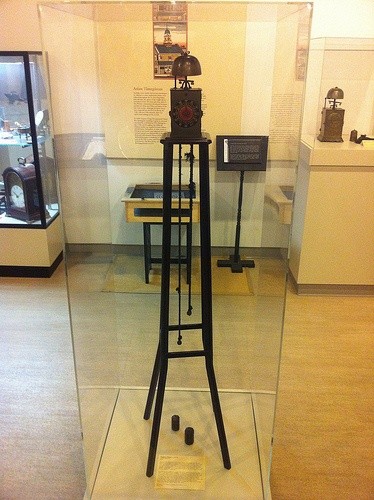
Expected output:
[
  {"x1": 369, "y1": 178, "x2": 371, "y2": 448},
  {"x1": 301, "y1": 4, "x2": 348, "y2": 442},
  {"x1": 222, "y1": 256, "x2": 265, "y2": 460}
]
[{"x1": 121, "y1": 183, "x2": 200, "y2": 284}]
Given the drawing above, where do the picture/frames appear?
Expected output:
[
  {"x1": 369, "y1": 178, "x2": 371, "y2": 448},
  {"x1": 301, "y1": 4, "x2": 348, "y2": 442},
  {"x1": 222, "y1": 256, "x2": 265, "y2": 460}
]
[{"x1": 152, "y1": 2, "x2": 189, "y2": 79}]
